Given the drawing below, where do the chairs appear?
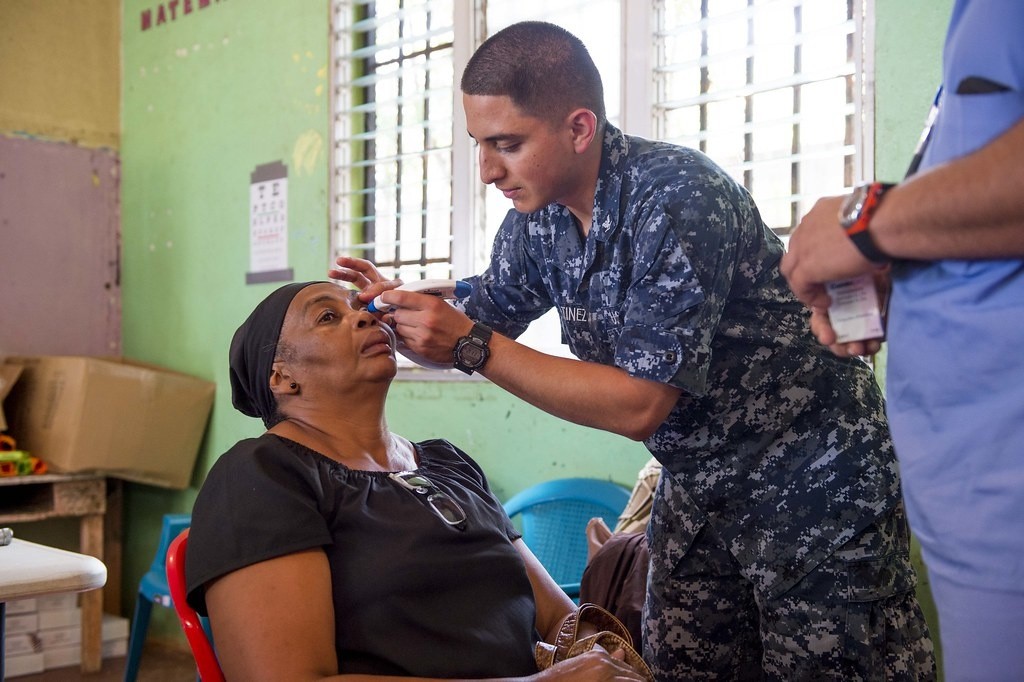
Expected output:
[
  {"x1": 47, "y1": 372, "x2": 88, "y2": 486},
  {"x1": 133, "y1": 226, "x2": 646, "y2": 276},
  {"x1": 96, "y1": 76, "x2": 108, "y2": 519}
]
[
  {"x1": 499, "y1": 478, "x2": 633, "y2": 606},
  {"x1": 121, "y1": 516, "x2": 211, "y2": 682},
  {"x1": 163, "y1": 528, "x2": 223, "y2": 682}
]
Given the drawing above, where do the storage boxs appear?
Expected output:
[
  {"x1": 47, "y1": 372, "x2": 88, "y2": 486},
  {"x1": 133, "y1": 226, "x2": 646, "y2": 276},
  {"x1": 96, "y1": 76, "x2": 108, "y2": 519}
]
[
  {"x1": 0, "y1": 353, "x2": 215, "y2": 487},
  {"x1": 1, "y1": 589, "x2": 130, "y2": 679}
]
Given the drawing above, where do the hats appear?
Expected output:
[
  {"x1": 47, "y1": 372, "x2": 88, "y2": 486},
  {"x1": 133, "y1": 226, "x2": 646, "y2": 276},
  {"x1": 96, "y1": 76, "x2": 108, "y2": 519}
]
[{"x1": 228, "y1": 281, "x2": 330, "y2": 425}]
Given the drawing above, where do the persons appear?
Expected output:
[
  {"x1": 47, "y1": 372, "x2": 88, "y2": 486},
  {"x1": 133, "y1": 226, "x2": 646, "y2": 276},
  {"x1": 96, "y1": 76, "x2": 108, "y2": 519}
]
[
  {"x1": 185, "y1": 280, "x2": 647, "y2": 682},
  {"x1": 780, "y1": 0, "x2": 1024, "y2": 682},
  {"x1": 330, "y1": 21, "x2": 936, "y2": 682}
]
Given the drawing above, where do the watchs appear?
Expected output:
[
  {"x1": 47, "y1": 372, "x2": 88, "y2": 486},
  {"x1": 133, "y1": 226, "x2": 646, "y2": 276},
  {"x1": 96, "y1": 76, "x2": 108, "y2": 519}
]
[
  {"x1": 836, "y1": 181, "x2": 896, "y2": 262},
  {"x1": 452, "y1": 321, "x2": 492, "y2": 376}
]
